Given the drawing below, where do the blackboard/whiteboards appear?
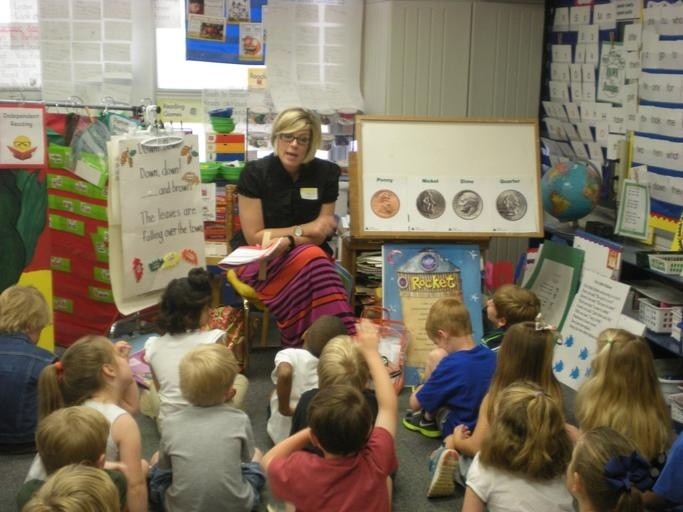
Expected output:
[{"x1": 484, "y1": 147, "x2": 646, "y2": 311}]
[{"x1": 355, "y1": 114, "x2": 544, "y2": 240}]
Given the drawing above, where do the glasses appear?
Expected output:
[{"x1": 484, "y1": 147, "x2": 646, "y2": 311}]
[{"x1": 279, "y1": 133, "x2": 311, "y2": 146}]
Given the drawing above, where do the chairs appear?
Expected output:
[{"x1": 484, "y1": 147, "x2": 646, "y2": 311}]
[{"x1": 222, "y1": 182, "x2": 271, "y2": 380}]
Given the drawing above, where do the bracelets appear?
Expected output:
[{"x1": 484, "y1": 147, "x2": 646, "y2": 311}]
[{"x1": 283, "y1": 235, "x2": 295, "y2": 253}]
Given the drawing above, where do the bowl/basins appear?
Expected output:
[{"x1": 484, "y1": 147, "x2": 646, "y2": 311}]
[
  {"x1": 200, "y1": 162, "x2": 219, "y2": 183},
  {"x1": 207, "y1": 106, "x2": 235, "y2": 133},
  {"x1": 220, "y1": 161, "x2": 245, "y2": 180}
]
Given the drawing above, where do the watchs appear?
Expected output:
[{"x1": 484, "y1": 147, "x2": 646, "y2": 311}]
[{"x1": 292, "y1": 224, "x2": 303, "y2": 237}]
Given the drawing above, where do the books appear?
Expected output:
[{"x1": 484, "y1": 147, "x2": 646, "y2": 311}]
[{"x1": 216, "y1": 237, "x2": 282, "y2": 277}]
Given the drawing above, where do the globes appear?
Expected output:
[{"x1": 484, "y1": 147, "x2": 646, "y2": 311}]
[{"x1": 541, "y1": 158, "x2": 602, "y2": 229}]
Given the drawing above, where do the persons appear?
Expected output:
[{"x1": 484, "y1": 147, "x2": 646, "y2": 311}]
[
  {"x1": 143, "y1": 267, "x2": 248, "y2": 437},
  {"x1": 17, "y1": 464, "x2": 120, "y2": 512},
  {"x1": 260, "y1": 318, "x2": 399, "y2": 511},
  {"x1": 459, "y1": 377, "x2": 574, "y2": 512},
  {"x1": 562, "y1": 426, "x2": 652, "y2": 511},
  {"x1": 288, "y1": 334, "x2": 378, "y2": 459},
  {"x1": 574, "y1": 327, "x2": 677, "y2": 487},
  {"x1": 266, "y1": 313, "x2": 347, "y2": 448},
  {"x1": 189, "y1": 0, "x2": 204, "y2": 15},
  {"x1": 22, "y1": 334, "x2": 148, "y2": 512},
  {"x1": 411, "y1": 283, "x2": 541, "y2": 395},
  {"x1": 642, "y1": 428, "x2": 683, "y2": 512},
  {"x1": 425, "y1": 319, "x2": 565, "y2": 499},
  {"x1": 148, "y1": 342, "x2": 264, "y2": 512},
  {"x1": 0, "y1": 283, "x2": 60, "y2": 457},
  {"x1": 224, "y1": 107, "x2": 399, "y2": 380},
  {"x1": 17, "y1": 405, "x2": 127, "y2": 511},
  {"x1": 401, "y1": 297, "x2": 497, "y2": 439}
]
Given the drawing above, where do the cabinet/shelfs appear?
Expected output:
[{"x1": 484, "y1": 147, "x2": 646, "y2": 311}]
[
  {"x1": 204, "y1": 131, "x2": 246, "y2": 163},
  {"x1": 617, "y1": 256, "x2": 682, "y2": 437}
]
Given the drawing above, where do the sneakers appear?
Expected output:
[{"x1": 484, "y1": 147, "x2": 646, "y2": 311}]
[
  {"x1": 402, "y1": 411, "x2": 442, "y2": 439},
  {"x1": 426, "y1": 449, "x2": 460, "y2": 499}
]
